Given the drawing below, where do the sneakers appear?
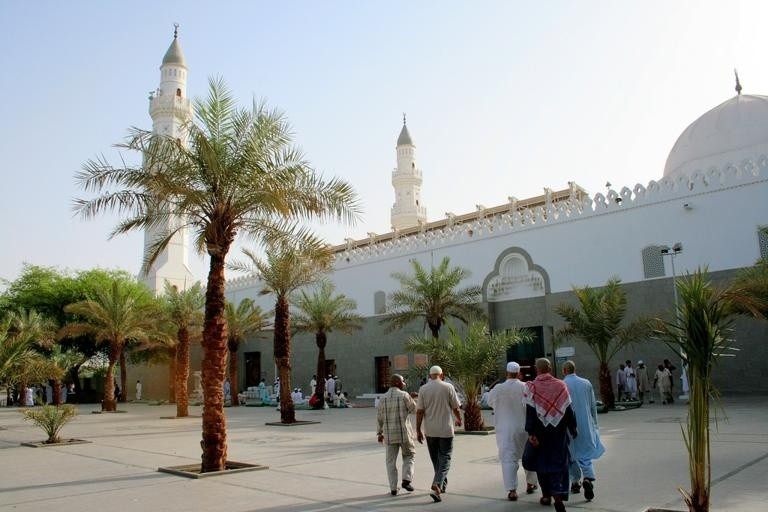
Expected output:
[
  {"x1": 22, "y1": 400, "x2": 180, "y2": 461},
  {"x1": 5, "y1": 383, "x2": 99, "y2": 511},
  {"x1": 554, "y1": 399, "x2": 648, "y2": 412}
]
[
  {"x1": 401, "y1": 481, "x2": 415, "y2": 492},
  {"x1": 390, "y1": 489, "x2": 399, "y2": 494}
]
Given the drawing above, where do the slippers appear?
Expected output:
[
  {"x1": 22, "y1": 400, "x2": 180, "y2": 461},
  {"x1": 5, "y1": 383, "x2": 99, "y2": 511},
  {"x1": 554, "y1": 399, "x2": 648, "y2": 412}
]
[{"x1": 430, "y1": 494, "x2": 442, "y2": 502}]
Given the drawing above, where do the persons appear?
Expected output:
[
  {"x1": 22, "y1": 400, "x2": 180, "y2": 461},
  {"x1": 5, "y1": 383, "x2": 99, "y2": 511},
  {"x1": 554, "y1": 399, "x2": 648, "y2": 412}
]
[
  {"x1": 679, "y1": 358, "x2": 691, "y2": 404},
  {"x1": 225, "y1": 378, "x2": 232, "y2": 395},
  {"x1": 112, "y1": 384, "x2": 121, "y2": 401},
  {"x1": 238, "y1": 390, "x2": 248, "y2": 405},
  {"x1": 561, "y1": 360, "x2": 606, "y2": 500},
  {"x1": 615, "y1": 357, "x2": 676, "y2": 404},
  {"x1": 136, "y1": 380, "x2": 142, "y2": 401},
  {"x1": 290, "y1": 374, "x2": 320, "y2": 411},
  {"x1": 325, "y1": 374, "x2": 352, "y2": 408},
  {"x1": 376, "y1": 373, "x2": 417, "y2": 495},
  {"x1": 259, "y1": 377, "x2": 282, "y2": 411},
  {"x1": 417, "y1": 365, "x2": 462, "y2": 502},
  {"x1": 522, "y1": 356, "x2": 576, "y2": 512},
  {"x1": 24, "y1": 380, "x2": 76, "y2": 406},
  {"x1": 487, "y1": 361, "x2": 538, "y2": 501}
]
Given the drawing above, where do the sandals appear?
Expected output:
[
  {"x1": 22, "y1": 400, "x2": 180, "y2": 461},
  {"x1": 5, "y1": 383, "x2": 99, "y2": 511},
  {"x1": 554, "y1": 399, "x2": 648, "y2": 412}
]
[
  {"x1": 508, "y1": 491, "x2": 518, "y2": 500},
  {"x1": 527, "y1": 484, "x2": 539, "y2": 493},
  {"x1": 571, "y1": 480, "x2": 594, "y2": 500},
  {"x1": 540, "y1": 495, "x2": 566, "y2": 511}
]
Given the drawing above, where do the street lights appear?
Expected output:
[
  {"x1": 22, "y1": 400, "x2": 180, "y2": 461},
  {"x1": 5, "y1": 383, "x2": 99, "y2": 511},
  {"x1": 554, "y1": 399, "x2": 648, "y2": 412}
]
[{"x1": 660, "y1": 240, "x2": 687, "y2": 402}]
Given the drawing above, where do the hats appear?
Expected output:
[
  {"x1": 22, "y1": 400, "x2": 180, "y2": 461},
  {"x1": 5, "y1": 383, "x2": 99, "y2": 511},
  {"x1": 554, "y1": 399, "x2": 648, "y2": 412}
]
[
  {"x1": 638, "y1": 360, "x2": 644, "y2": 364},
  {"x1": 429, "y1": 366, "x2": 442, "y2": 375},
  {"x1": 507, "y1": 361, "x2": 520, "y2": 373}
]
